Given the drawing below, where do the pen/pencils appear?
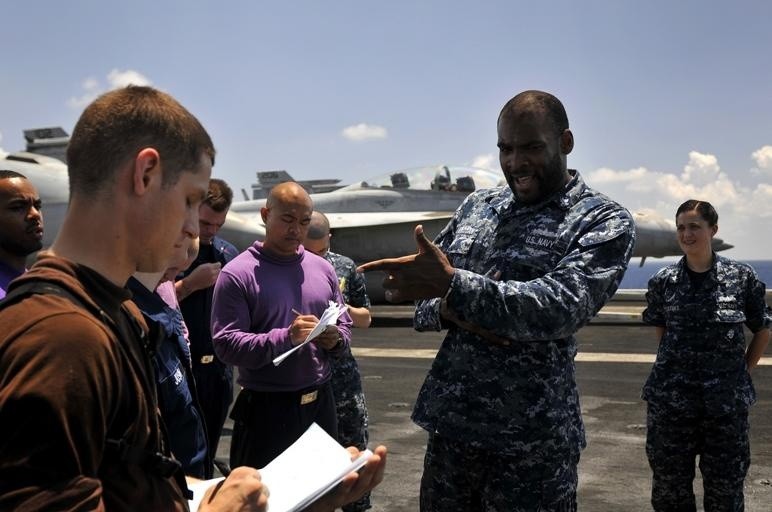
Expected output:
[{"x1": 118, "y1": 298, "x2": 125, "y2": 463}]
[
  {"x1": 292, "y1": 309, "x2": 302, "y2": 316},
  {"x1": 212, "y1": 459, "x2": 231, "y2": 478}
]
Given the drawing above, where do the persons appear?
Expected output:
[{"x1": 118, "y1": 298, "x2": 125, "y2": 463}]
[
  {"x1": 1, "y1": 81, "x2": 387, "y2": 511},
  {"x1": 638, "y1": 199, "x2": 771, "y2": 512},
  {"x1": 355, "y1": 85, "x2": 637, "y2": 512}
]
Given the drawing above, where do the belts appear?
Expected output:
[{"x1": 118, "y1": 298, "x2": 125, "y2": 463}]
[
  {"x1": 241, "y1": 382, "x2": 328, "y2": 408},
  {"x1": 191, "y1": 351, "x2": 217, "y2": 366}
]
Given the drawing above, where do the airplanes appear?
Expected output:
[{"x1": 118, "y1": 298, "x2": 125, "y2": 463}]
[
  {"x1": 21, "y1": 124, "x2": 734, "y2": 308},
  {"x1": 0, "y1": 150, "x2": 268, "y2": 272}
]
